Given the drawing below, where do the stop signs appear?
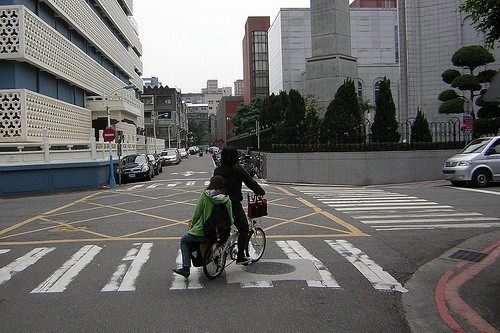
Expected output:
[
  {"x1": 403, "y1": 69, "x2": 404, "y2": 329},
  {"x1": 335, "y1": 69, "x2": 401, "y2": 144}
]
[{"x1": 103, "y1": 129, "x2": 115, "y2": 142}]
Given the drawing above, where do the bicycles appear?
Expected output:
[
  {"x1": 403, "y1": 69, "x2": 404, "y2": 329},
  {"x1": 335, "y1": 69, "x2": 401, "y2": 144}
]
[
  {"x1": 211, "y1": 150, "x2": 263, "y2": 179},
  {"x1": 202, "y1": 192, "x2": 267, "y2": 279}
]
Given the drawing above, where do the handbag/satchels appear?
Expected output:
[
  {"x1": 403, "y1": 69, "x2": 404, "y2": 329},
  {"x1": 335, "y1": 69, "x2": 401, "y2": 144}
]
[{"x1": 248, "y1": 197, "x2": 268, "y2": 219}]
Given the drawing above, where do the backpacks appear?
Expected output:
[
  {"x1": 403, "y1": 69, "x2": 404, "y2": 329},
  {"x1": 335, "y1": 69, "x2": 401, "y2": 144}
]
[{"x1": 202, "y1": 200, "x2": 232, "y2": 244}]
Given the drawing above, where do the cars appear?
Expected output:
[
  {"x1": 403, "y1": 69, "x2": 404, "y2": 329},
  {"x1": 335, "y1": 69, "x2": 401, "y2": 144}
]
[
  {"x1": 207, "y1": 146, "x2": 220, "y2": 154},
  {"x1": 145, "y1": 154, "x2": 161, "y2": 176},
  {"x1": 178, "y1": 148, "x2": 188, "y2": 159},
  {"x1": 115, "y1": 154, "x2": 155, "y2": 184},
  {"x1": 159, "y1": 148, "x2": 182, "y2": 165},
  {"x1": 442, "y1": 136, "x2": 500, "y2": 188},
  {"x1": 188, "y1": 146, "x2": 200, "y2": 154}
]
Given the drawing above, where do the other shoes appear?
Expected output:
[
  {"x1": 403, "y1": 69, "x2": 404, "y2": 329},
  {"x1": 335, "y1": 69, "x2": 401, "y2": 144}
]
[
  {"x1": 235, "y1": 253, "x2": 253, "y2": 265},
  {"x1": 172, "y1": 267, "x2": 190, "y2": 278}
]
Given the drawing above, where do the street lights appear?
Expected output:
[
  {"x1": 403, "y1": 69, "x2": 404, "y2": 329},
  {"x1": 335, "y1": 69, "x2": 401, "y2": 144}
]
[
  {"x1": 168, "y1": 121, "x2": 180, "y2": 149},
  {"x1": 153, "y1": 112, "x2": 169, "y2": 152},
  {"x1": 107, "y1": 84, "x2": 137, "y2": 187}
]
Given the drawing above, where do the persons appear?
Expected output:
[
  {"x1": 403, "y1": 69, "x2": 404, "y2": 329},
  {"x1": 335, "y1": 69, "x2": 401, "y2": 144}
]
[
  {"x1": 213, "y1": 146, "x2": 264, "y2": 266},
  {"x1": 172, "y1": 176, "x2": 233, "y2": 278}
]
